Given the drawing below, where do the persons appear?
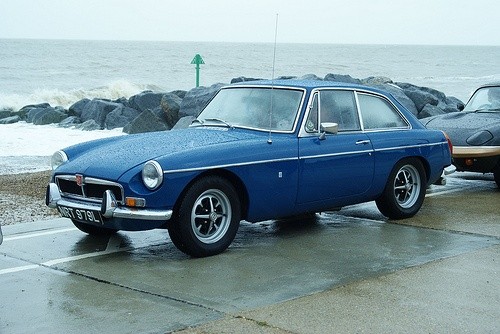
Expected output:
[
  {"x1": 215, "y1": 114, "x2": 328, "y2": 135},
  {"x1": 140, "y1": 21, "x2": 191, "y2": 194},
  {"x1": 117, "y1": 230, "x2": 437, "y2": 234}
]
[{"x1": 289, "y1": 89, "x2": 345, "y2": 131}]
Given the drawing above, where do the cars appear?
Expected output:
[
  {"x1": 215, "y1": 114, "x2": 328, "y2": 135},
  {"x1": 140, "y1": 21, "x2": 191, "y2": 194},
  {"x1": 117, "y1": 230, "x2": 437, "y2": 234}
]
[
  {"x1": 42, "y1": 79, "x2": 451, "y2": 261},
  {"x1": 414, "y1": 81, "x2": 500, "y2": 187}
]
